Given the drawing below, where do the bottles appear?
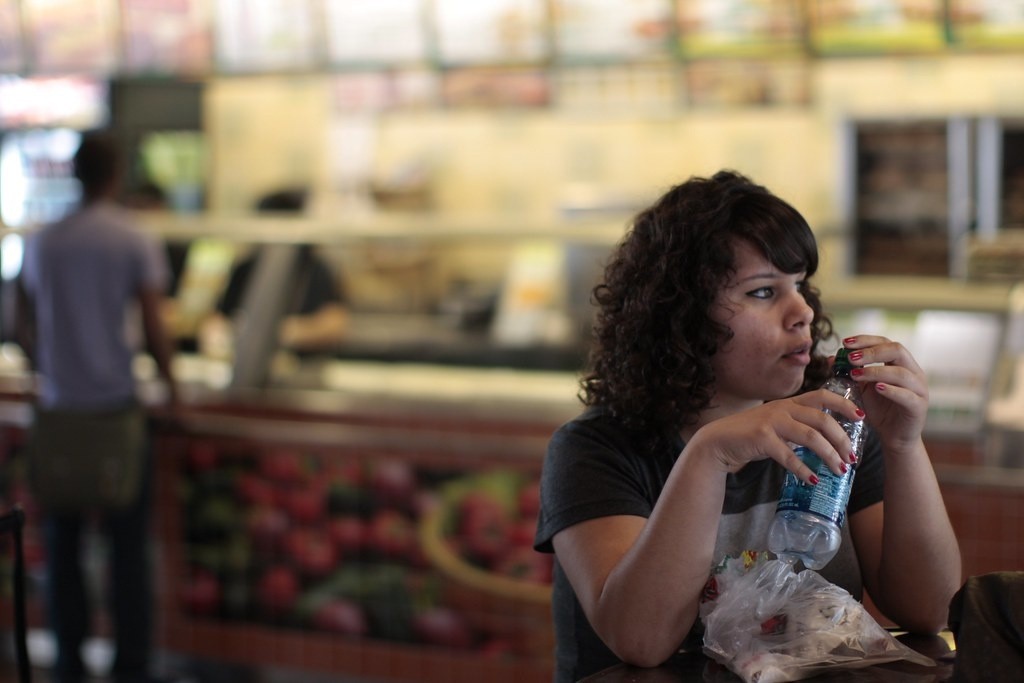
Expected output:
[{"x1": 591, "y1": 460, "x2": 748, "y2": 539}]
[{"x1": 767, "y1": 348, "x2": 870, "y2": 570}]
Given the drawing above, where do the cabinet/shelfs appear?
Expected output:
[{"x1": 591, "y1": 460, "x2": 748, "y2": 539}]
[
  {"x1": 836, "y1": 112, "x2": 1024, "y2": 276},
  {"x1": 0, "y1": 221, "x2": 1024, "y2": 683}
]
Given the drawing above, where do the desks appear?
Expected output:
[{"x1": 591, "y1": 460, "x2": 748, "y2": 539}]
[{"x1": 556, "y1": 626, "x2": 956, "y2": 683}]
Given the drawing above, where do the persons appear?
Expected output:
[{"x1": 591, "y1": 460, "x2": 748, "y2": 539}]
[
  {"x1": 250, "y1": 293, "x2": 267, "y2": 296},
  {"x1": 116, "y1": 181, "x2": 349, "y2": 360},
  {"x1": 0, "y1": 126, "x2": 183, "y2": 683},
  {"x1": 532, "y1": 172, "x2": 959, "y2": 682}
]
